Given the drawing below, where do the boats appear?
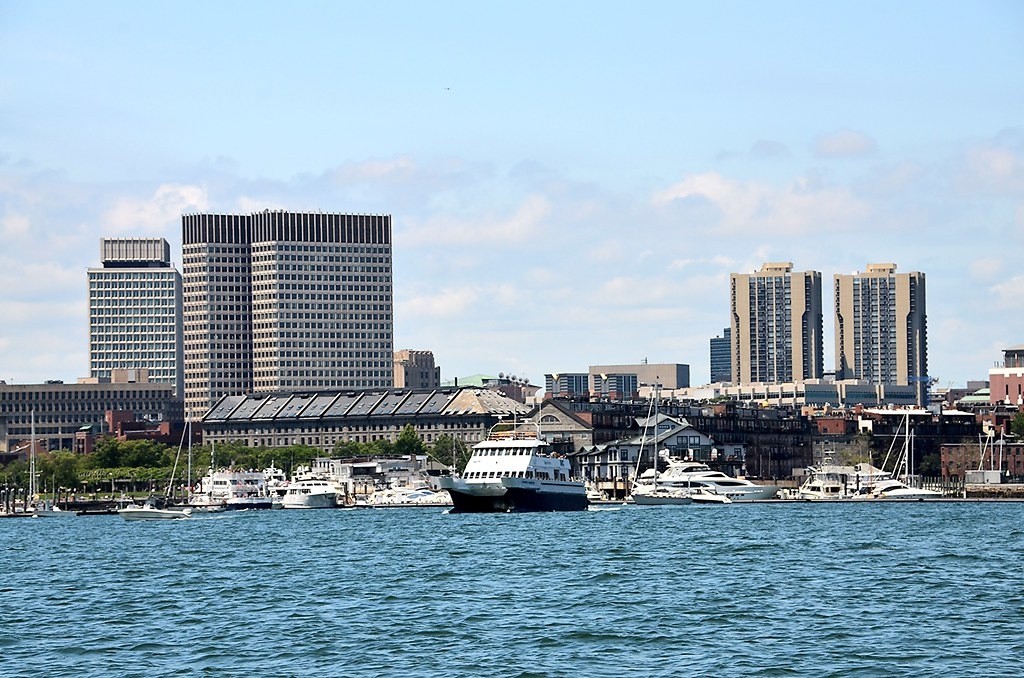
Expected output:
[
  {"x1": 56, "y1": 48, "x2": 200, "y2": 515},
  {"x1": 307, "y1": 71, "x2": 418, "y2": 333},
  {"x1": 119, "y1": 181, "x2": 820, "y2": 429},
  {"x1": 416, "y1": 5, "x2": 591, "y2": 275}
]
[
  {"x1": 640, "y1": 456, "x2": 783, "y2": 500},
  {"x1": 144, "y1": 408, "x2": 345, "y2": 510},
  {"x1": 773, "y1": 403, "x2": 942, "y2": 499},
  {"x1": 117, "y1": 504, "x2": 192, "y2": 521},
  {"x1": 438, "y1": 402, "x2": 590, "y2": 513}
]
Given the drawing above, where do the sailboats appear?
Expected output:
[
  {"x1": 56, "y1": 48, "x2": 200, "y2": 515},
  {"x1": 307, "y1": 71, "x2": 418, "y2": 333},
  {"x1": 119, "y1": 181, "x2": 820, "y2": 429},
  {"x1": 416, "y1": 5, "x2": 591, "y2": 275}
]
[
  {"x1": 6, "y1": 410, "x2": 77, "y2": 517},
  {"x1": 631, "y1": 371, "x2": 732, "y2": 506}
]
